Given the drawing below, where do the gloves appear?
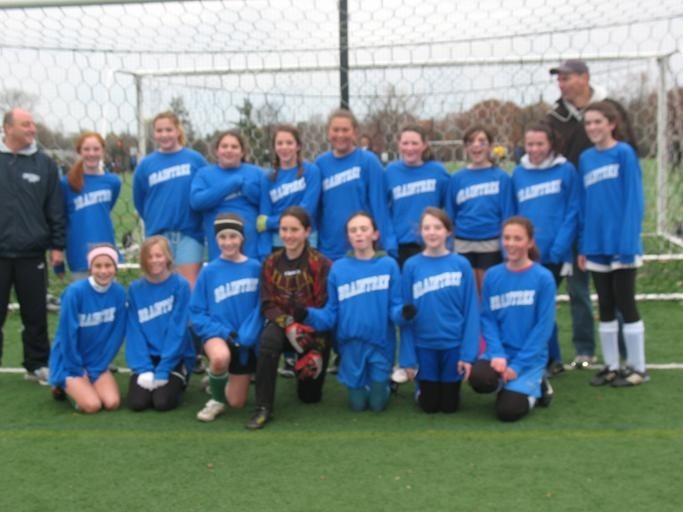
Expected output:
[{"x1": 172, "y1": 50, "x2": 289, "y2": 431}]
[
  {"x1": 153, "y1": 379, "x2": 168, "y2": 389},
  {"x1": 136, "y1": 371, "x2": 154, "y2": 392},
  {"x1": 284, "y1": 321, "x2": 315, "y2": 355},
  {"x1": 293, "y1": 351, "x2": 324, "y2": 384}
]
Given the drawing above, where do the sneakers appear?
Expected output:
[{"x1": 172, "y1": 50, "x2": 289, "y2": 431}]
[
  {"x1": 24, "y1": 372, "x2": 49, "y2": 386},
  {"x1": 197, "y1": 399, "x2": 224, "y2": 421},
  {"x1": 561, "y1": 356, "x2": 593, "y2": 371},
  {"x1": 535, "y1": 376, "x2": 554, "y2": 410},
  {"x1": 328, "y1": 360, "x2": 342, "y2": 379},
  {"x1": 612, "y1": 370, "x2": 651, "y2": 387},
  {"x1": 588, "y1": 371, "x2": 616, "y2": 387},
  {"x1": 245, "y1": 408, "x2": 273, "y2": 430},
  {"x1": 280, "y1": 360, "x2": 294, "y2": 380}
]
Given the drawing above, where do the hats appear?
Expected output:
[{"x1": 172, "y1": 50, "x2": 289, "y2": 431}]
[{"x1": 548, "y1": 56, "x2": 590, "y2": 76}]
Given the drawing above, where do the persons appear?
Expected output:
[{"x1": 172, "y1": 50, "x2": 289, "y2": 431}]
[
  {"x1": 383, "y1": 124, "x2": 452, "y2": 273},
  {"x1": 540, "y1": 59, "x2": 640, "y2": 370},
  {"x1": 59, "y1": 130, "x2": 123, "y2": 281},
  {"x1": 121, "y1": 233, "x2": 191, "y2": 413},
  {"x1": 256, "y1": 126, "x2": 323, "y2": 253},
  {"x1": 502, "y1": 126, "x2": 583, "y2": 376},
  {"x1": 445, "y1": 126, "x2": 513, "y2": 301},
  {"x1": 189, "y1": 131, "x2": 265, "y2": 275},
  {"x1": 576, "y1": 97, "x2": 653, "y2": 385},
  {"x1": 130, "y1": 110, "x2": 207, "y2": 291},
  {"x1": 313, "y1": 110, "x2": 400, "y2": 261},
  {"x1": 245, "y1": 205, "x2": 333, "y2": 432},
  {"x1": 47, "y1": 242, "x2": 127, "y2": 416},
  {"x1": 393, "y1": 206, "x2": 475, "y2": 415},
  {"x1": 292, "y1": 212, "x2": 418, "y2": 419},
  {"x1": 0, "y1": 105, "x2": 64, "y2": 386},
  {"x1": 471, "y1": 215, "x2": 558, "y2": 423},
  {"x1": 185, "y1": 211, "x2": 263, "y2": 425}
]
